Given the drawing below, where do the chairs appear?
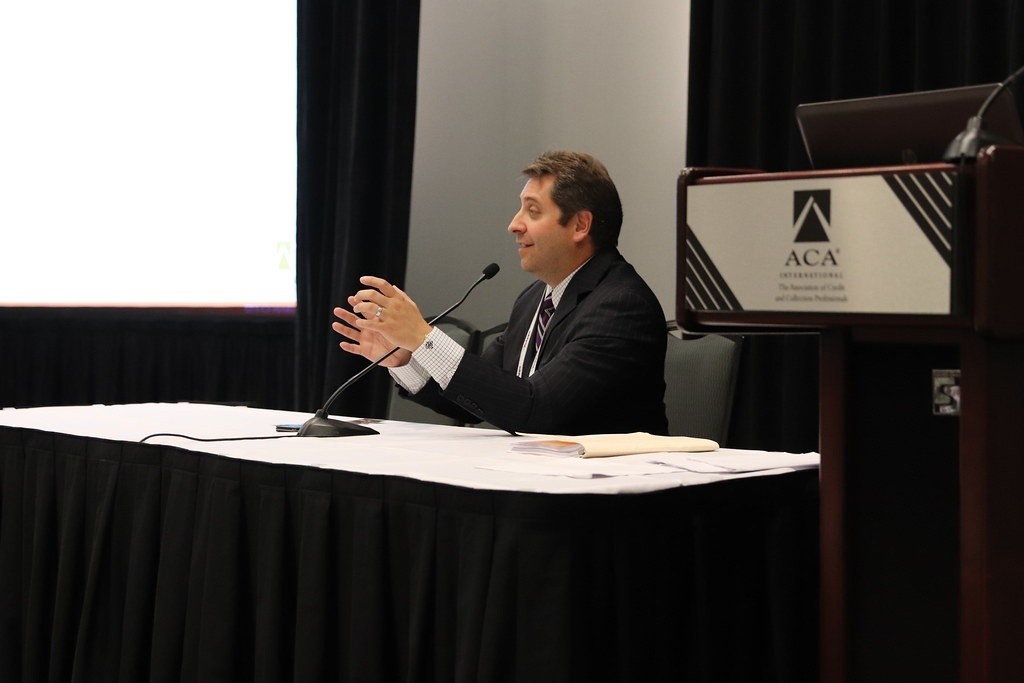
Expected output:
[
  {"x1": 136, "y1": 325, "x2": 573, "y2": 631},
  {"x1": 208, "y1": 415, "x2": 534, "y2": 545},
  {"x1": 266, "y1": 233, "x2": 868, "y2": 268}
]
[
  {"x1": 388, "y1": 317, "x2": 510, "y2": 434},
  {"x1": 662, "y1": 322, "x2": 741, "y2": 446}
]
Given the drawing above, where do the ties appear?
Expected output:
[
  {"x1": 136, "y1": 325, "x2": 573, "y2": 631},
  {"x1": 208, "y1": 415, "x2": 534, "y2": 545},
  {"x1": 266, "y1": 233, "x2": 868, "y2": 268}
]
[{"x1": 535, "y1": 291, "x2": 555, "y2": 355}]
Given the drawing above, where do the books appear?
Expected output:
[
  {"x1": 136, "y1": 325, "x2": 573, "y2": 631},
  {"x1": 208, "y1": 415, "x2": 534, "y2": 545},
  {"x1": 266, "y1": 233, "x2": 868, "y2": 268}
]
[{"x1": 509, "y1": 431, "x2": 719, "y2": 458}]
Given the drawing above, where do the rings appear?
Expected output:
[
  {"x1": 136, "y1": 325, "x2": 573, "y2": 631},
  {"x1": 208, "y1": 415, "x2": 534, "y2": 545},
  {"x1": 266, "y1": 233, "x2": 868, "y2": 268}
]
[{"x1": 375, "y1": 306, "x2": 383, "y2": 317}]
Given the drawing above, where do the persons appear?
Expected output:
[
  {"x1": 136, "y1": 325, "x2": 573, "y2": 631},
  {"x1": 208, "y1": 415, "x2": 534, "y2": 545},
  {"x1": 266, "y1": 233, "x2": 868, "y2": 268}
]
[{"x1": 332, "y1": 149, "x2": 670, "y2": 436}]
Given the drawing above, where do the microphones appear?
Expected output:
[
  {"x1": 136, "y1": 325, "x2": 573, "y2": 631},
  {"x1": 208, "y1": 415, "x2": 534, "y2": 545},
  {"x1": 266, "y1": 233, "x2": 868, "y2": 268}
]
[{"x1": 300, "y1": 262, "x2": 501, "y2": 437}]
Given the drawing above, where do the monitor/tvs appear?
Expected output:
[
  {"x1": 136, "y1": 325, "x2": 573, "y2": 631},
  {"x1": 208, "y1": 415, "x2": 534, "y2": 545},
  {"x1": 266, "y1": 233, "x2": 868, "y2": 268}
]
[{"x1": 797, "y1": 84, "x2": 1021, "y2": 172}]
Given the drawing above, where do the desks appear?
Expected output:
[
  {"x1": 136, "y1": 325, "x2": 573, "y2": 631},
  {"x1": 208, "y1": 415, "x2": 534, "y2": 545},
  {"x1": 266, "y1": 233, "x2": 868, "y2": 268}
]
[{"x1": 0, "y1": 401, "x2": 858, "y2": 683}]
